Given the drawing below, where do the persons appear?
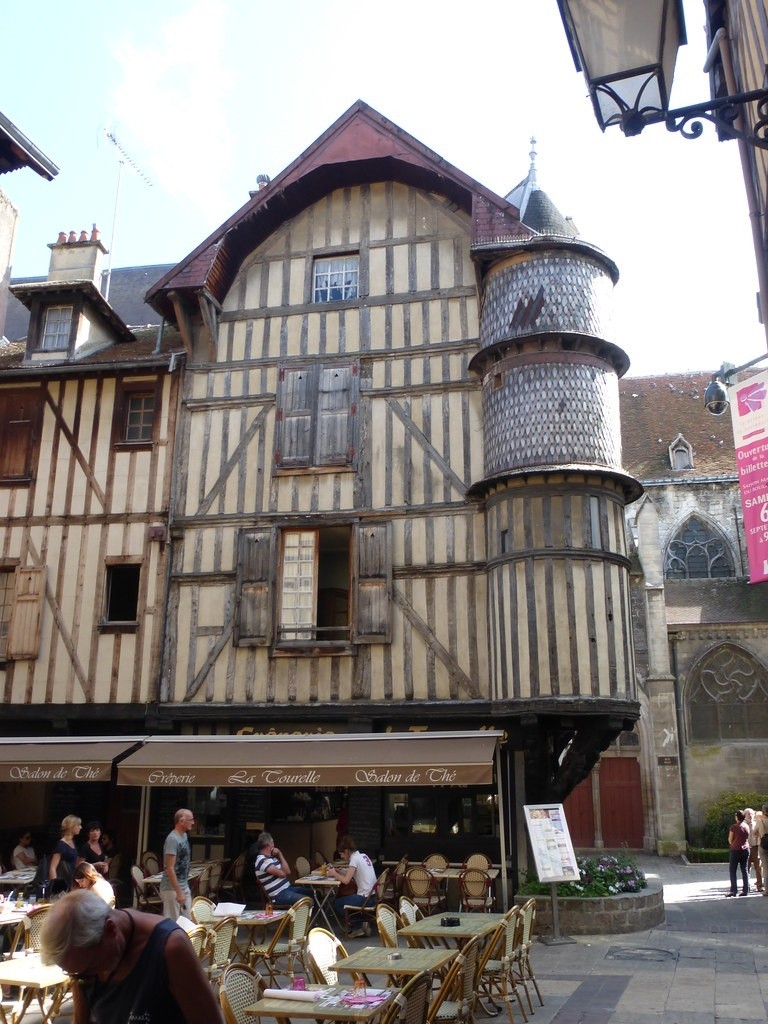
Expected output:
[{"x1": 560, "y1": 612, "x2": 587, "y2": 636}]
[
  {"x1": 250, "y1": 832, "x2": 321, "y2": 906},
  {"x1": 323, "y1": 835, "x2": 378, "y2": 938},
  {"x1": 725, "y1": 804, "x2": 768, "y2": 898},
  {"x1": 159, "y1": 809, "x2": 195, "y2": 923},
  {"x1": 37, "y1": 889, "x2": 224, "y2": 1024},
  {"x1": 13, "y1": 813, "x2": 122, "y2": 894}
]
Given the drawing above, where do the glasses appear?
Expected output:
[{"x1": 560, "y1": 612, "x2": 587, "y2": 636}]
[
  {"x1": 102, "y1": 836, "x2": 107, "y2": 841},
  {"x1": 62, "y1": 948, "x2": 101, "y2": 979},
  {"x1": 23, "y1": 836, "x2": 30, "y2": 840}
]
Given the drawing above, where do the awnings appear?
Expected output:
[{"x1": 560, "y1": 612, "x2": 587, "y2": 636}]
[
  {"x1": 0, "y1": 741, "x2": 139, "y2": 781},
  {"x1": 116, "y1": 735, "x2": 496, "y2": 788}
]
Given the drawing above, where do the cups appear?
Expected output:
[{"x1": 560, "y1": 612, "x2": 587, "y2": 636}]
[
  {"x1": 29, "y1": 894, "x2": 36, "y2": 904},
  {"x1": 354, "y1": 980, "x2": 366, "y2": 998},
  {"x1": 322, "y1": 865, "x2": 327, "y2": 874},
  {"x1": 292, "y1": 977, "x2": 305, "y2": 991},
  {"x1": 265, "y1": 903, "x2": 273, "y2": 916}
]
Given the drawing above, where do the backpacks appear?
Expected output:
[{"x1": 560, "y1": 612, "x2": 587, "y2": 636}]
[{"x1": 758, "y1": 819, "x2": 768, "y2": 850}]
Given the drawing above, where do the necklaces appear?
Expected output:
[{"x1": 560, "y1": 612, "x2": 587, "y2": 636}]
[{"x1": 104, "y1": 909, "x2": 134, "y2": 986}]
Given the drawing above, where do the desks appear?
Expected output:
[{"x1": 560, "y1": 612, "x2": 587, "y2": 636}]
[{"x1": 0, "y1": 861, "x2": 509, "y2": 1024}]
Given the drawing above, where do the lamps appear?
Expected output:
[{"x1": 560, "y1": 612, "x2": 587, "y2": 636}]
[{"x1": 556, "y1": 1, "x2": 768, "y2": 151}]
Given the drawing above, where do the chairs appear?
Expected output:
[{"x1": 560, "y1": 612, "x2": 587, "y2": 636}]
[
  {"x1": 105, "y1": 848, "x2": 545, "y2": 1024},
  {"x1": 4, "y1": 905, "x2": 54, "y2": 961}
]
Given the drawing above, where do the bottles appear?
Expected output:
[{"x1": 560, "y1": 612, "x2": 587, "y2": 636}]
[{"x1": 17, "y1": 892, "x2": 24, "y2": 908}]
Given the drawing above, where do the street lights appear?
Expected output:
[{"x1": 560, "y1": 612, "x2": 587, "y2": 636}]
[
  {"x1": 555, "y1": 0, "x2": 766, "y2": 347},
  {"x1": 104, "y1": 159, "x2": 128, "y2": 303}
]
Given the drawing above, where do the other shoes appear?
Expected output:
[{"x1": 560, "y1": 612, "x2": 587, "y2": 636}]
[
  {"x1": 763, "y1": 893, "x2": 768, "y2": 896},
  {"x1": 725, "y1": 892, "x2": 736, "y2": 897},
  {"x1": 348, "y1": 929, "x2": 365, "y2": 938},
  {"x1": 739, "y1": 892, "x2": 747, "y2": 896},
  {"x1": 362, "y1": 921, "x2": 372, "y2": 936},
  {"x1": 758, "y1": 888, "x2": 763, "y2": 891}
]
